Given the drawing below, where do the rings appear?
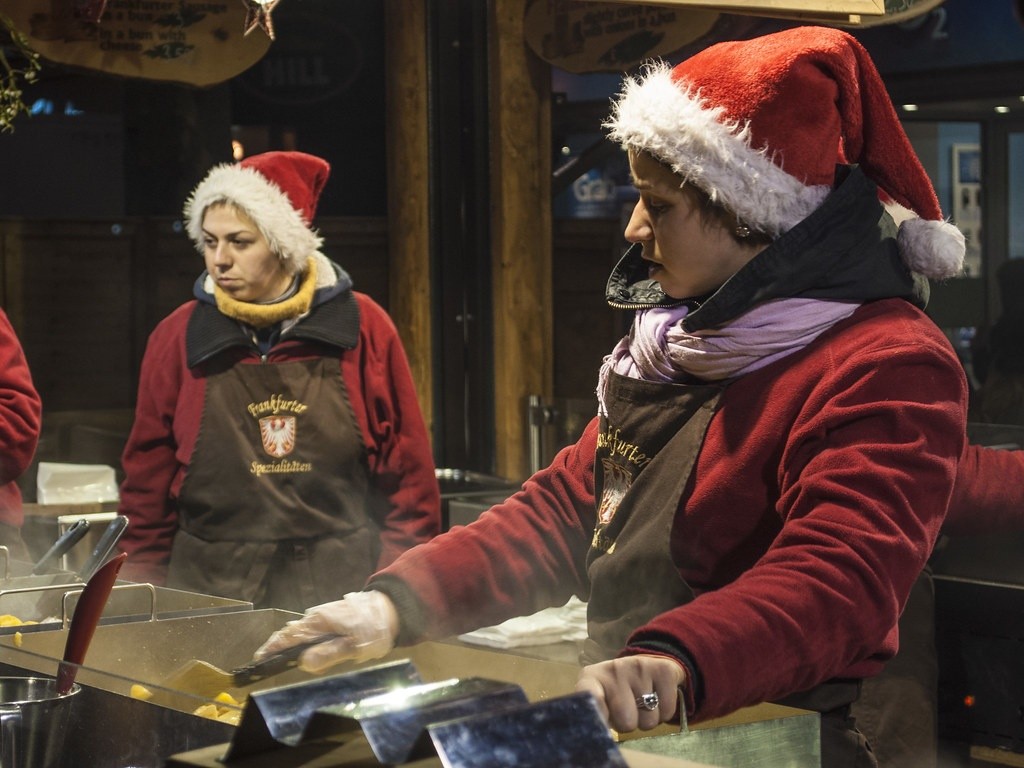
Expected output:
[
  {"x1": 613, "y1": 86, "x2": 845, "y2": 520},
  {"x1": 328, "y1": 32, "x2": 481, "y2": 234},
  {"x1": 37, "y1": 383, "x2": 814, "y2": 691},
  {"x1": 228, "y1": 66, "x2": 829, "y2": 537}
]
[{"x1": 636, "y1": 691, "x2": 659, "y2": 710}]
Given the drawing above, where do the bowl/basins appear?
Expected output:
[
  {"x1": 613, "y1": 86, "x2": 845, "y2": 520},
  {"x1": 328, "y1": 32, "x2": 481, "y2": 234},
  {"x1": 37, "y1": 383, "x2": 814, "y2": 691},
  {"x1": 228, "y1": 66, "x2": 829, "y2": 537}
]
[{"x1": 0, "y1": 677, "x2": 81, "y2": 768}]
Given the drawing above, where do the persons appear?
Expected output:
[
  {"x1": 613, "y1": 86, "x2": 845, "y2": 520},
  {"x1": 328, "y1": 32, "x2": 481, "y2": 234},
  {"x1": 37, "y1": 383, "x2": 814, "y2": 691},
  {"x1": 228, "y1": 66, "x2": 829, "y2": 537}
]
[
  {"x1": 121, "y1": 152, "x2": 441, "y2": 613},
  {"x1": 0, "y1": 307, "x2": 42, "y2": 564},
  {"x1": 254, "y1": 26, "x2": 1024, "y2": 768}
]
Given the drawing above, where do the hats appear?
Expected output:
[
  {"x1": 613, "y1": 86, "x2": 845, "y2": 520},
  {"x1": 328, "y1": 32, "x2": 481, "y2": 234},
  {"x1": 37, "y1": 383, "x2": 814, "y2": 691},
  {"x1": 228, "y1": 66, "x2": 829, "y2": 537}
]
[
  {"x1": 604, "y1": 26, "x2": 966, "y2": 279},
  {"x1": 185, "y1": 151, "x2": 330, "y2": 272}
]
